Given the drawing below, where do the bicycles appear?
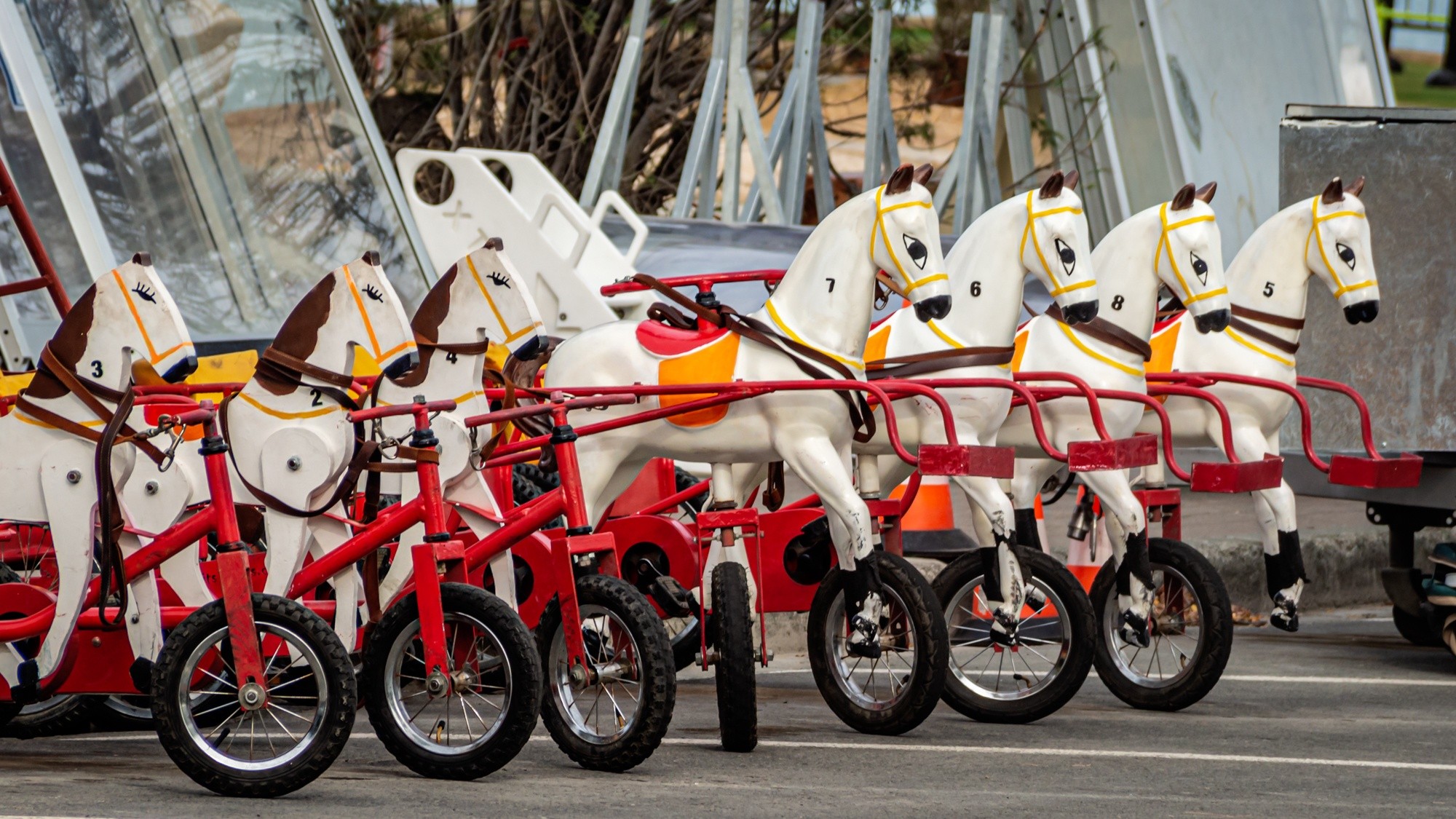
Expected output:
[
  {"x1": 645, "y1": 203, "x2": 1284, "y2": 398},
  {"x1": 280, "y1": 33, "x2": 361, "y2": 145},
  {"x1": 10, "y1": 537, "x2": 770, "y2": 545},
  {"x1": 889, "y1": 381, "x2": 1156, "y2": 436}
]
[{"x1": 0, "y1": 161, "x2": 1379, "y2": 798}]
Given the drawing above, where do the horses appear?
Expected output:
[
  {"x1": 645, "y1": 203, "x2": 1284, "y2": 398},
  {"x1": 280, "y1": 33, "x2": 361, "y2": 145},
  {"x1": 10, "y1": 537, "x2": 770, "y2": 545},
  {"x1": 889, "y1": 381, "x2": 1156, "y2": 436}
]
[
  {"x1": 859, "y1": 181, "x2": 1232, "y2": 648},
  {"x1": 348, "y1": 237, "x2": 549, "y2": 628},
  {"x1": 159, "y1": 250, "x2": 421, "y2": 653},
  {"x1": 502, "y1": 164, "x2": 953, "y2": 599},
  {"x1": 701, "y1": 172, "x2": 1099, "y2": 655},
  {"x1": 1011, "y1": 176, "x2": 1379, "y2": 632},
  {"x1": 0, "y1": 250, "x2": 193, "y2": 687}
]
